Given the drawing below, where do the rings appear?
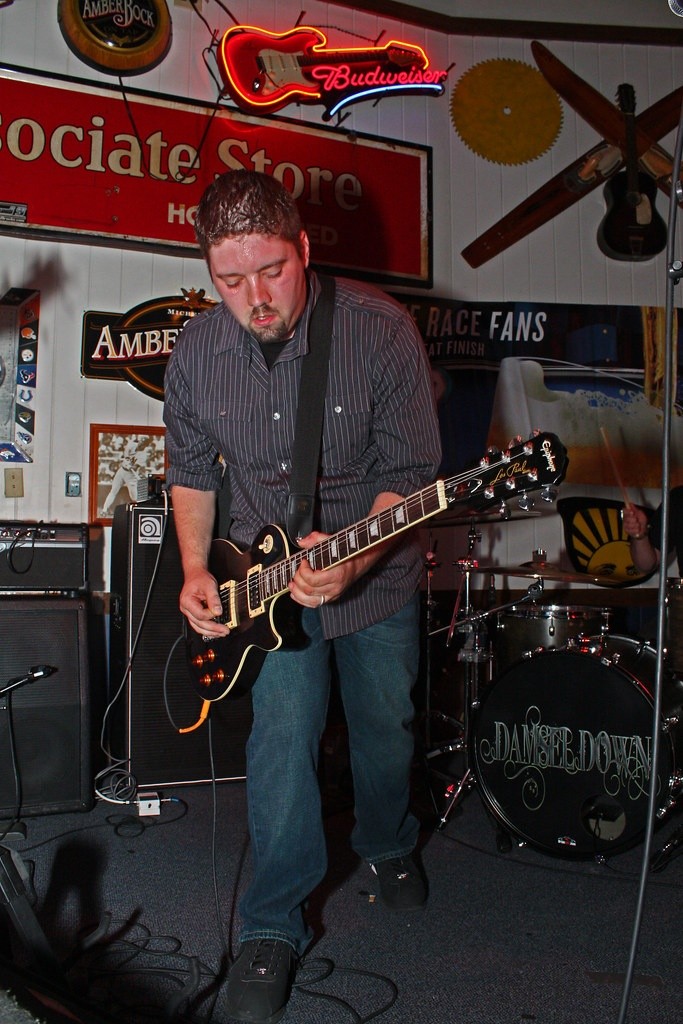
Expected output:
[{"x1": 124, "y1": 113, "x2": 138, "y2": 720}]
[{"x1": 319, "y1": 596, "x2": 325, "y2": 608}]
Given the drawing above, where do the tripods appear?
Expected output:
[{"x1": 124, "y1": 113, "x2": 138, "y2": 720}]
[{"x1": 416, "y1": 520, "x2": 545, "y2": 833}]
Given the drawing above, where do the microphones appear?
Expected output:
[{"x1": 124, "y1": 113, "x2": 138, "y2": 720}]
[{"x1": 28, "y1": 666, "x2": 53, "y2": 677}]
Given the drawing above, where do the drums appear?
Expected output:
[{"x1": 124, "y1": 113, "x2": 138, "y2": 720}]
[
  {"x1": 464, "y1": 630, "x2": 683, "y2": 864},
  {"x1": 495, "y1": 603, "x2": 610, "y2": 652}
]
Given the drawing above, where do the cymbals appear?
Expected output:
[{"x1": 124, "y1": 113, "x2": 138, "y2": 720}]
[{"x1": 458, "y1": 559, "x2": 625, "y2": 589}]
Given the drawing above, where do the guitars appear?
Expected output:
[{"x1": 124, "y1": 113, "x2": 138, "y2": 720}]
[{"x1": 184, "y1": 426, "x2": 572, "y2": 708}]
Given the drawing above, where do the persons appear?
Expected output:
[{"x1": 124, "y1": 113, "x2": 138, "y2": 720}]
[
  {"x1": 161, "y1": 169, "x2": 443, "y2": 1024},
  {"x1": 622, "y1": 482, "x2": 683, "y2": 578}
]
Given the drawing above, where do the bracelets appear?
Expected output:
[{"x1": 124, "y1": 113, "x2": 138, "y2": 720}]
[{"x1": 629, "y1": 531, "x2": 648, "y2": 540}]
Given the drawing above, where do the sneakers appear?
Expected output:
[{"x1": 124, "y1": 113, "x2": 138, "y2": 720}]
[
  {"x1": 369, "y1": 853, "x2": 426, "y2": 912},
  {"x1": 225, "y1": 939, "x2": 294, "y2": 1024}
]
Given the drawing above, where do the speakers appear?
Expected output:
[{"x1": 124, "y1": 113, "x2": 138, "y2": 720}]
[
  {"x1": 0, "y1": 593, "x2": 96, "y2": 818},
  {"x1": 125, "y1": 502, "x2": 275, "y2": 789}
]
